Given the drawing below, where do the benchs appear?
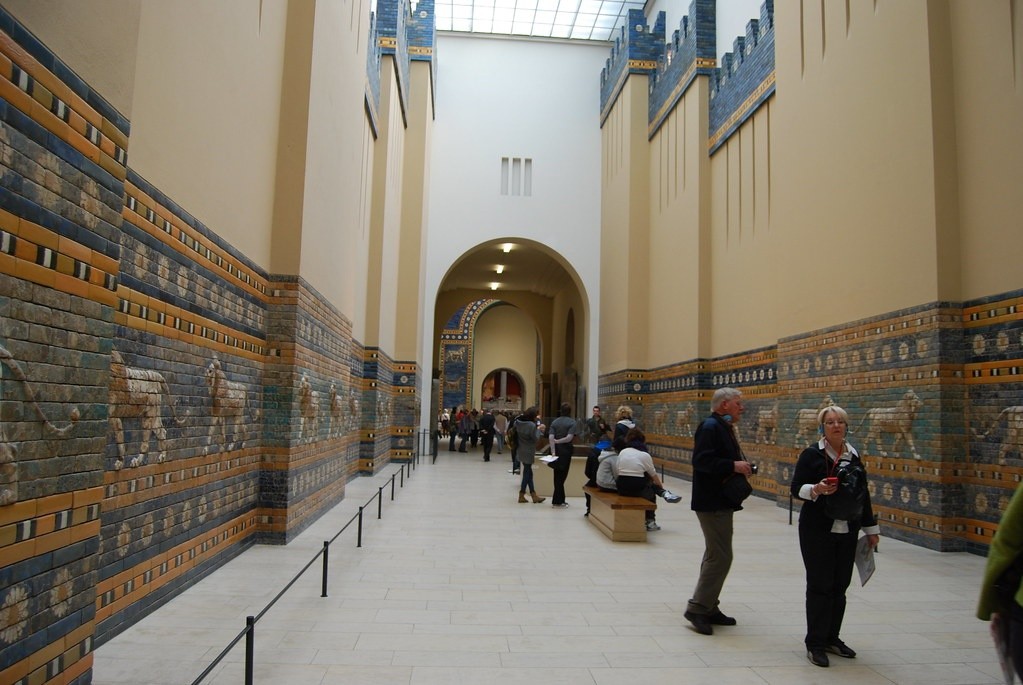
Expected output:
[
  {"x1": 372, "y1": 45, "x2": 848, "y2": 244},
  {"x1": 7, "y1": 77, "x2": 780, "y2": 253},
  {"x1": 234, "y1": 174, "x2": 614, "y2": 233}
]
[{"x1": 581, "y1": 487, "x2": 658, "y2": 542}]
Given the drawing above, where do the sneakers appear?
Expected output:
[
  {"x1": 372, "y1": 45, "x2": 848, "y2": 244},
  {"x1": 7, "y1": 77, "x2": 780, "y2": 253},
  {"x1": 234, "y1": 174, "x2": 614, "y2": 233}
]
[
  {"x1": 807, "y1": 646, "x2": 830, "y2": 667},
  {"x1": 823, "y1": 638, "x2": 856, "y2": 657}
]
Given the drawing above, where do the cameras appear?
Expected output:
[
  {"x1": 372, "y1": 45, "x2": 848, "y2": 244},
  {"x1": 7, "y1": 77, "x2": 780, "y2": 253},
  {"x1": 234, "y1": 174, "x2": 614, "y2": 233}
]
[{"x1": 748, "y1": 463, "x2": 758, "y2": 474}]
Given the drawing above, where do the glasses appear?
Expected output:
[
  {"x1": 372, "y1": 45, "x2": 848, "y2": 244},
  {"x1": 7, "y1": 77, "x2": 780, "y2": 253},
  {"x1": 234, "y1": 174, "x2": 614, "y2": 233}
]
[{"x1": 823, "y1": 420, "x2": 845, "y2": 426}]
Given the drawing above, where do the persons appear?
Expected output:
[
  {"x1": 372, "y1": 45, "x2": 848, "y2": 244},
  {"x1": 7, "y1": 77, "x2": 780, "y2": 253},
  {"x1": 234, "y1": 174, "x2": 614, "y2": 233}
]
[
  {"x1": 614, "y1": 405, "x2": 636, "y2": 454},
  {"x1": 584, "y1": 406, "x2": 612, "y2": 447},
  {"x1": 584, "y1": 430, "x2": 682, "y2": 531},
  {"x1": 513, "y1": 407, "x2": 546, "y2": 503},
  {"x1": 977, "y1": 481, "x2": 1023, "y2": 685},
  {"x1": 508, "y1": 412, "x2": 521, "y2": 473},
  {"x1": 438, "y1": 406, "x2": 543, "y2": 454},
  {"x1": 790, "y1": 406, "x2": 881, "y2": 667},
  {"x1": 683, "y1": 387, "x2": 755, "y2": 635},
  {"x1": 548, "y1": 403, "x2": 576, "y2": 508},
  {"x1": 479, "y1": 407, "x2": 495, "y2": 461}
]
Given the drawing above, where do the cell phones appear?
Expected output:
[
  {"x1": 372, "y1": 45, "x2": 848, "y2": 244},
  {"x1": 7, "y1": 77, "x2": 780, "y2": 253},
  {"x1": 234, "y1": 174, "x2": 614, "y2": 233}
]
[{"x1": 827, "y1": 478, "x2": 838, "y2": 485}]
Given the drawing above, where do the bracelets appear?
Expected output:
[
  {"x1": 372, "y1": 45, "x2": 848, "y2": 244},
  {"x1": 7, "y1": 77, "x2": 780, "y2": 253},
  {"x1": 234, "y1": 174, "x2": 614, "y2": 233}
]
[{"x1": 811, "y1": 487, "x2": 818, "y2": 498}]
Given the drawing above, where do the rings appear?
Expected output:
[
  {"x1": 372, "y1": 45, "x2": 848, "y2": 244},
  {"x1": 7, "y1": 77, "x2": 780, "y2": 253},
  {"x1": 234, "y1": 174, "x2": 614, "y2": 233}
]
[{"x1": 826, "y1": 492, "x2": 828, "y2": 494}]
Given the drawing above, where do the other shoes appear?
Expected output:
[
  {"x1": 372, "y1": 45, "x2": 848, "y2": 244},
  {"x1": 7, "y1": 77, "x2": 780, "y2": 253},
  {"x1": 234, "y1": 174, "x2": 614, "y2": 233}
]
[
  {"x1": 685, "y1": 611, "x2": 713, "y2": 635},
  {"x1": 708, "y1": 612, "x2": 737, "y2": 627}
]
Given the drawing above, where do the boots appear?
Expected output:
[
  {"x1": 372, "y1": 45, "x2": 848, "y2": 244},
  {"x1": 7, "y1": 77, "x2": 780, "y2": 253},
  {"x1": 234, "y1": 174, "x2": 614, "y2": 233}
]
[
  {"x1": 661, "y1": 491, "x2": 682, "y2": 503},
  {"x1": 647, "y1": 518, "x2": 660, "y2": 530},
  {"x1": 531, "y1": 493, "x2": 546, "y2": 503},
  {"x1": 518, "y1": 491, "x2": 529, "y2": 503}
]
